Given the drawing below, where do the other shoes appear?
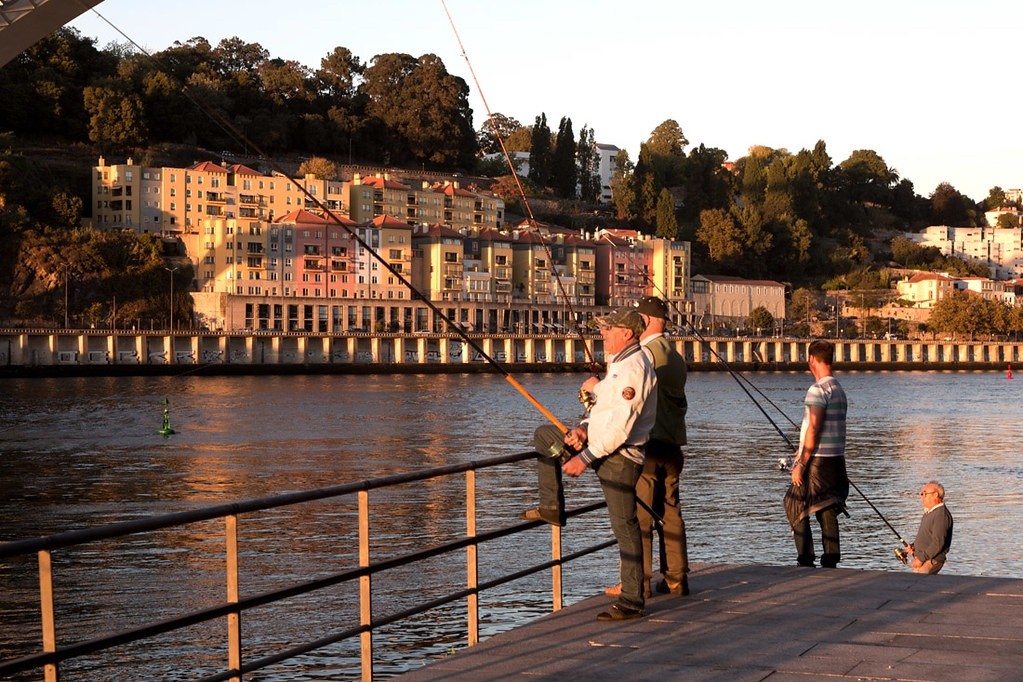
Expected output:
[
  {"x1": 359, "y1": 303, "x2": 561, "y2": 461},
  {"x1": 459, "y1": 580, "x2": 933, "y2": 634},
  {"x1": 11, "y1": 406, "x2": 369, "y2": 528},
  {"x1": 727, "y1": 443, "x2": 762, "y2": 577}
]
[{"x1": 786, "y1": 562, "x2": 817, "y2": 570}]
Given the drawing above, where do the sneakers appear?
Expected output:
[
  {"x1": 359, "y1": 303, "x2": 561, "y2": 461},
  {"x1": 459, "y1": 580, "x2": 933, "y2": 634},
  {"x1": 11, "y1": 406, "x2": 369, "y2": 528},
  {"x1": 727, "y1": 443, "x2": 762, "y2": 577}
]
[
  {"x1": 655, "y1": 577, "x2": 690, "y2": 596},
  {"x1": 605, "y1": 581, "x2": 651, "y2": 599}
]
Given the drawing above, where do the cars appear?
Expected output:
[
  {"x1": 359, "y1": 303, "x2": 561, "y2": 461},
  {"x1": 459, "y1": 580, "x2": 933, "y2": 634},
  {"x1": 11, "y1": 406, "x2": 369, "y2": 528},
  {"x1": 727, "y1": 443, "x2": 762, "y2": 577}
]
[
  {"x1": 195, "y1": 327, "x2": 210, "y2": 331},
  {"x1": 268, "y1": 327, "x2": 284, "y2": 330},
  {"x1": 256, "y1": 328, "x2": 268, "y2": 331},
  {"x1": 288, "y1": 327, "x2": 308, "y2": 332},
  {"x1": 413, "y1": 328, "x2": 430, "y2": 333}
]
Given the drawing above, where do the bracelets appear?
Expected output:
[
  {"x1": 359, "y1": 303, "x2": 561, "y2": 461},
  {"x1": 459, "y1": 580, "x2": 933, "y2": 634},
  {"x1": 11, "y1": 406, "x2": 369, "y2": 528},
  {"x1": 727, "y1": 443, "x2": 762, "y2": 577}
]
[{"x1": 797, "y1": 461, "x2": 807, "y2": 468}]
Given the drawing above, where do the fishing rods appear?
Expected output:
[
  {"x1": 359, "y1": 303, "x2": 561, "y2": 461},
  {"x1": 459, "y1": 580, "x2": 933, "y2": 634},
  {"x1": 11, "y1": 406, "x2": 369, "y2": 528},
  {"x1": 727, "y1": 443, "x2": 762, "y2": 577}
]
[
  {"x1": 439, "y1": 0, "x2": 601, "y2": 381},
  {"x1": 87, "y1": 3, "x2": 668, "y2": 527},
  {"x1": 601, "y1": 232, "x2": 852, "y2": 522},
  {"x1": 669, "y1": 319, "x2": 914, "y2": 566}
]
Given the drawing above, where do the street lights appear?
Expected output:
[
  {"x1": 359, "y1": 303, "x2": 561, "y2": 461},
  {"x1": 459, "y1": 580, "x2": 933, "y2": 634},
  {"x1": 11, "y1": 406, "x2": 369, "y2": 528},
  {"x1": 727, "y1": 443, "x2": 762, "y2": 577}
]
[
  {"x1": 164, "y1": 267, "x2": 179, "y2": 331},
  {"x1": 60, "y1": 261, "x2": 77, "y2": 329}
]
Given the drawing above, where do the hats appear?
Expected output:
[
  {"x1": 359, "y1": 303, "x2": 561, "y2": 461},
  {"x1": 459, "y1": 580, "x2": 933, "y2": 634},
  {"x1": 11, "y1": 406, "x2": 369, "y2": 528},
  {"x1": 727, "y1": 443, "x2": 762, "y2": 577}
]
[
  {"x1": 594, "y1": 307, "x2": 646, "y2": 340},
  {"x1": 637, "y1": 297, "x2": 671, "y2": 321}
]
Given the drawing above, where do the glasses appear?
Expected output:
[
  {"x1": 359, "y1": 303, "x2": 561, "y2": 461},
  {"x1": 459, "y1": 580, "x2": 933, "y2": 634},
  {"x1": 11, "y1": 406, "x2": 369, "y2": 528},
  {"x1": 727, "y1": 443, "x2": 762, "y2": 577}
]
[
  {"x1": 595, "y1": 324, "x2": 630, "y2": 330},
  {"x1": 919, "y1": 492, "x2": 935, "y2": 497}
]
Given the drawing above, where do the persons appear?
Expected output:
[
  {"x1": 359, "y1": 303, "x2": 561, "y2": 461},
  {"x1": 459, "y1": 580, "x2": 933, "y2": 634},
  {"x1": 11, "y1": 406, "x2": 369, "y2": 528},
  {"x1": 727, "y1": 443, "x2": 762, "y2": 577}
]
[
  {"x1": 782, "y1": 339, "x2": 849, "y2": 568},
  {"x1": 904, "y1": 480, "x2": 953, "y2": 574},
  {"x1": 580, "y1": 295, "x2": 691, "y2": 596},
  {"x1": 517, "y1": 305, "x2": 659, "y2": 621}
]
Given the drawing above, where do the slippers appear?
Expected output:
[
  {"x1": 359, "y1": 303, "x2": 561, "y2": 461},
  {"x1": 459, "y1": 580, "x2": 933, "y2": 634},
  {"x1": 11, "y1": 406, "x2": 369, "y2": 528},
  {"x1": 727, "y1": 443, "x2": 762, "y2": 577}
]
[
  {"x1": 596, "y1": 605, "x2": 642, "y2": 621},
  {"x1": 518, "y1": 509, "x2": 566, "y2": 526}
]
